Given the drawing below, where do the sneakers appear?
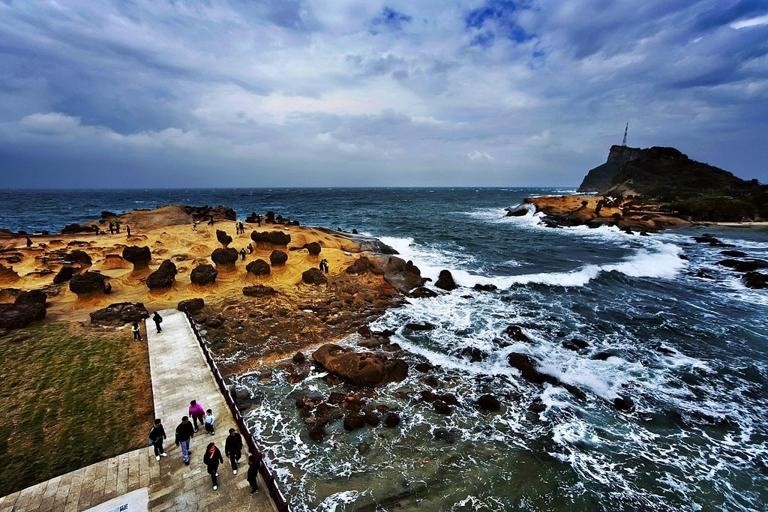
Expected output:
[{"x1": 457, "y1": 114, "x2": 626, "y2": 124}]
[
  {"x1": 156, "y1": 427, "x2": 215, "y2": 467},
  {"x1": 212, "y1": 468, "x2": 260, "y2": 497}
]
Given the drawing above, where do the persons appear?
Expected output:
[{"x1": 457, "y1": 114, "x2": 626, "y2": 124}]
[
  {"x1": 109, "y1": 221, "x2": 114, "y2": 234},
  {"x1": 24, "y1": 235, "x2": 32, "y2": 247},
  {"x1": 204, "y1": 442, "x2": 223, "y2": 490},
  {"x1": 148, "y1": 418, "x2": 167, "y2": 461},
  {"x1": 248, "y1": 450, "x2": 262, "y2": 495},
  {"x1": 203, "y1": 408, "x2": 216, "y2": 434},
  {"x1": 189, "y1": 400, "x2": 205, "y2": 432},
  {"x1": 208, "y1": 216, "x2": 213, "y2": 226},
  {"x1": 225, "y1": 428, "x2": 243, "y2": 474},
  {"x1": 132, "y1": 321, "x2": 143, "y2": 342},
  {"x1": 153, "y1": 311, "x2": 163, "y2": 333},
  {"x1": 240, "y1": 243, "x2": 253, "y2": 259},
  {"x1": 116, "y1": 223, "x2": 119, "y2": 233},
  {"x1": 175, "y1": 416, "x2": 194, "y2": 464},
  {"x1": 238, "y1": 221, "x2": 245, "y2": 234},
  {"x1": 236, "y1": 223, "x2": 239, "y2": 234},
  {"x1": 320, "y1": 259, "x2": 329, "y2": 273},
  {"x1": 192, "y1": 222, "x2": 195, "y2": 230},
  {"x1": 127, "y1": 225, "x2": 130, "y2": 235}
]
